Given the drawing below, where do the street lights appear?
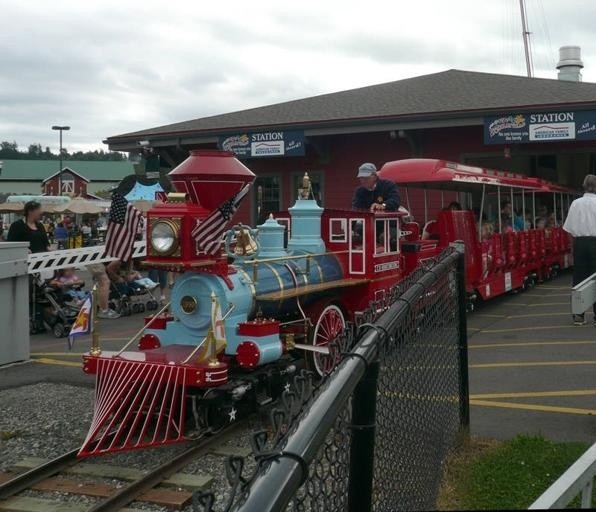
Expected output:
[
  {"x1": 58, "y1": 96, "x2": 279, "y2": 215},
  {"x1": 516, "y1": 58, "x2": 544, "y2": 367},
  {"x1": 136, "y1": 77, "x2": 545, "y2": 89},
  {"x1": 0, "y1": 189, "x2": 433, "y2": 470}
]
[{"x1": 52, "y1": 126, "x2": 70, "y2": 196}]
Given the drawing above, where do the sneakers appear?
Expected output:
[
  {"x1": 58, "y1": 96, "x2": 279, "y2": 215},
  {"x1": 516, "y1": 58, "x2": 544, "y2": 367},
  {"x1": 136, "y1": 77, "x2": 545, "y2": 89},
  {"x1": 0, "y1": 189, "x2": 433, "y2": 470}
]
[
  {"x1": 573, "y1": 314, "x2": 588, "y2": 325},
  {"x1": 157, "y1": 281, "x2": 175, "y2": 302},
  {"x1": 95, "y1": 307, "x2": 121, "y2": 319}
]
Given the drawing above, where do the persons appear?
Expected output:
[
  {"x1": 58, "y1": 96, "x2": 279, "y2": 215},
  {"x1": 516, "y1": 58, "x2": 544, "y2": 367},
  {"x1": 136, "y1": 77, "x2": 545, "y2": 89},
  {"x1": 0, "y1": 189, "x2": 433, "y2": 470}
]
[
  {"x1": 352, "y1": 161, "x2": 401, "y2": 244},
  {"x1": 422, "y1": 200, "x2": 562, "y2": 253},
  {"x1": 562, "y1": 175, "x2": 596, "y2": 325},
  {"x1": 0, "y1": 201, "x2": 180, "y2": 319}
]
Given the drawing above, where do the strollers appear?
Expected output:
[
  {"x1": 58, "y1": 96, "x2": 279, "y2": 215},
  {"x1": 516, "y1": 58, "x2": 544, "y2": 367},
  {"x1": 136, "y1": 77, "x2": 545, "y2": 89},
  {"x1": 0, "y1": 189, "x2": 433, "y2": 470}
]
[
  {"x1": 92, "y1": 262, "x2": 159, "y2": 316},
  {"x1": 31, "y1": 272, "x2": 92, "y2": 339}
]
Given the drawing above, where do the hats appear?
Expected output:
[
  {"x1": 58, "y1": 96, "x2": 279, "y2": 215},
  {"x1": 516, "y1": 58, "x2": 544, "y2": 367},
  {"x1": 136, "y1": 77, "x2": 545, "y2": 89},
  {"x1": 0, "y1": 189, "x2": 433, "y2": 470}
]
[{"x1": 356, "y1": 163, "x2": 378, "y2": 179}]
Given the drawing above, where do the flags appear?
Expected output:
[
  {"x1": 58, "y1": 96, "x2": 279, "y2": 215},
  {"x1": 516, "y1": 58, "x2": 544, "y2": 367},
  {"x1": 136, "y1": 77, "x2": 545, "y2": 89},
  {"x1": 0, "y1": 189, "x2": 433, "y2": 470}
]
[
  {"x1": 104, "y1": 191, "x2": 141, "y2": 263},
  {"x1": 190, "y1": 184, "x2": 250, "y2": 256}
]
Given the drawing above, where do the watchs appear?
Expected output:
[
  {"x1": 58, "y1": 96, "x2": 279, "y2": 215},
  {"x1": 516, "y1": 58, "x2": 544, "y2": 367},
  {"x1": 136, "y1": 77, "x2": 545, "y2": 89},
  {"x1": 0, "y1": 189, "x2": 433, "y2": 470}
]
[{"x1": 382, "y1": 203, "x2": 386, "y2": 209}]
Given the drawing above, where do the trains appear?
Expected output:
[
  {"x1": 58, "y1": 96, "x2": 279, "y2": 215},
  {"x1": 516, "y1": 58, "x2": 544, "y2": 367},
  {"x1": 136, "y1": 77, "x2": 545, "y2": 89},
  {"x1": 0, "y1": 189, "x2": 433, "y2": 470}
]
[{"x1": 75, "y1": 148, "x2": 583, "y2": 460}]
